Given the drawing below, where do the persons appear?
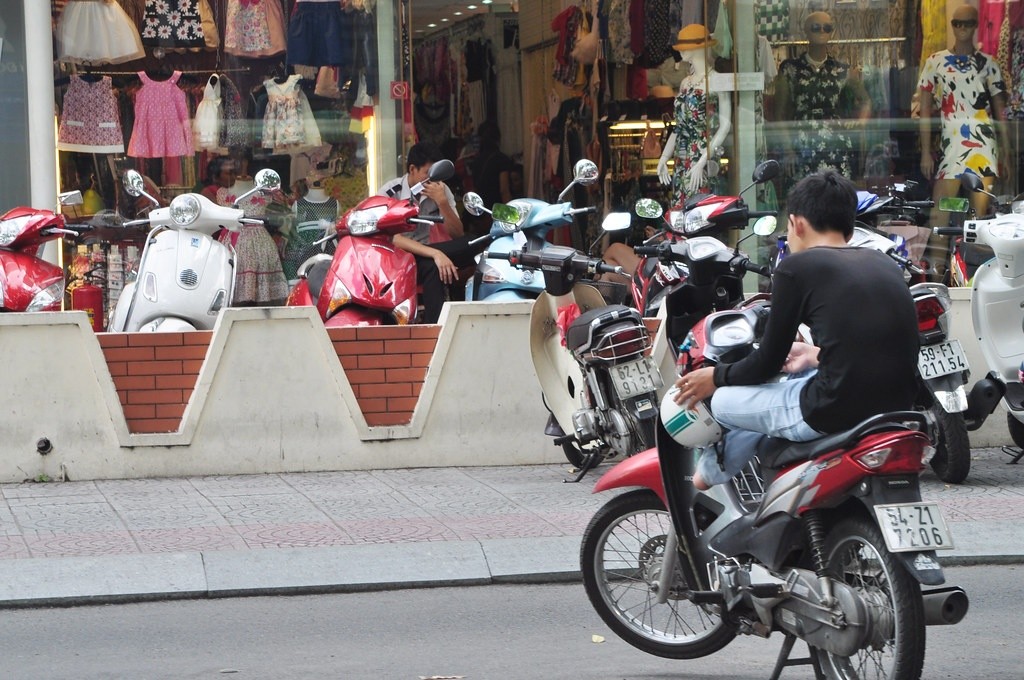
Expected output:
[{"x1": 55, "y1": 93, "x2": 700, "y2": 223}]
[
  {"x1": 378, "y1": 141, "x2": 482, "y2": 323},
  {"x1": 673, "y1": 172, "x2": 935, "y2": 492},
  {"x1": 594, "y1": 226, "x2": 663, "y2": 304},
  {"x1": 656, "y1": 25, "x2": 732, "y2": 241},
  {"x1": 216, "y1": 176, "x2": 289, "y2": 303},
  {"x1": 460, "y1": 122, "x2": 523, "y2": 235},
  {"x1": 917, "y1": 4, "x2": 1013, "y2": 284},
  {"x1": 197, "y1": 156, "x2": 237, "y2": 201},
  {"x1": 283, "y1": 188, "x2": 339, "y2": 281},
  {"x1": 774, "y1": 11, "x2": 873, "y2": 231}
]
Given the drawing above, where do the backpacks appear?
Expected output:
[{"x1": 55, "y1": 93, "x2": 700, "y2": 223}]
[{"x1": 639, "y1": 120, "x2": 661, "y2": 160}]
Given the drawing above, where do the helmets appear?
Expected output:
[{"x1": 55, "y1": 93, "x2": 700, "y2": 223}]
[{"x1": 659, "y1": 373, "x2": 723, "y2": 452}]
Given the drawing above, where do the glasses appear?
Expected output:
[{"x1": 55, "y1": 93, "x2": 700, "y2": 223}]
[
  {"x1": 810, "y1": 23, "x2": 833, "y2": 33},
  {"x1": 951, "y1": 19, "x2": 976, "y2": 28}
]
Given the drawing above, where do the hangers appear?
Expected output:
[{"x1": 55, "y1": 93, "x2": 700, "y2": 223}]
[
  {"x1": 74, "y1": 59, "x2": 103, "y2": 84},
  {"x1": 414, "y1": 12, "x2": 489, "y2": 57},
  {"x1": 206, "y1": 60, "x2": 229, "y2": 87},
  {"x1": 252, "y1": 66, "x2": 304, "y2": 93},
  {"x1": 138, "y1": 56, "x2": 175, "y2": 82}
]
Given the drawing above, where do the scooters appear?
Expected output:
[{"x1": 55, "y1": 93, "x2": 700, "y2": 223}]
[
  {"x1": 630, "y1": 160, "x2": 778, "y2": 315},
  {"x1": 631, "y1": 197, "x2": 778, "y2": 414},
  {"x1": 0, "y1": 188, "x2": 91, "y2": 314},
  {"x1": 462, "y1": 159, "x2": 598, "y2": 302},
  {"x1": 296, "y1": 160, "x2": 456, "y2": 327},
  {"x1": 932, "y1": 172, "x2": 1024, "y2": 464},
  {"x1": 846, "y1": 219, "x2": 972, "y2": 483},
  {"x1": 778, "y1": 191, "x2": 911, "y2": 273},
  {"x1": 106, "y1": 167, "x2": 281, "y2": 332}
]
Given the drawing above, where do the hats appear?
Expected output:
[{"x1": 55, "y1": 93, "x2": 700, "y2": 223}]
[
  {"x1": 648, "y1": 85, "x2": 675, "y2": 99},
  {"x1": 671, "y1": 24, "x2": 717, "y2": 51}
]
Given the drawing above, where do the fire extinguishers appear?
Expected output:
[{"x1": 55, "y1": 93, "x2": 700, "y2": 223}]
[{"x1": 71, "y1": 266, "x2": 106, "y2": 333}]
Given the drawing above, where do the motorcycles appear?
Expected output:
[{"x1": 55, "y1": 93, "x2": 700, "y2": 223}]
[
  {"x1": 481, "y1": 203, "x2": 667, "y2": 483},
  {"x1": 579, "y1": 300, "x2": 968, "y2": 680}
]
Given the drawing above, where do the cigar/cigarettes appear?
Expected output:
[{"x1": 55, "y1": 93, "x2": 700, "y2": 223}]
[{"x1": 669, "y1": 388, "x2": 680, "y2": 395}]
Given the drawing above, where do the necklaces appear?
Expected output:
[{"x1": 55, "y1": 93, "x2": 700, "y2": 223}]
[
  {"x1": 806, "y1": 53, "x2": 827, "y2": 73},
  {"x1": 687, "y1": 67, "x2": 713, "y2": 88},
  {"x1": 953, "y1": 45, "x2": 974, "y2": 54}
]
